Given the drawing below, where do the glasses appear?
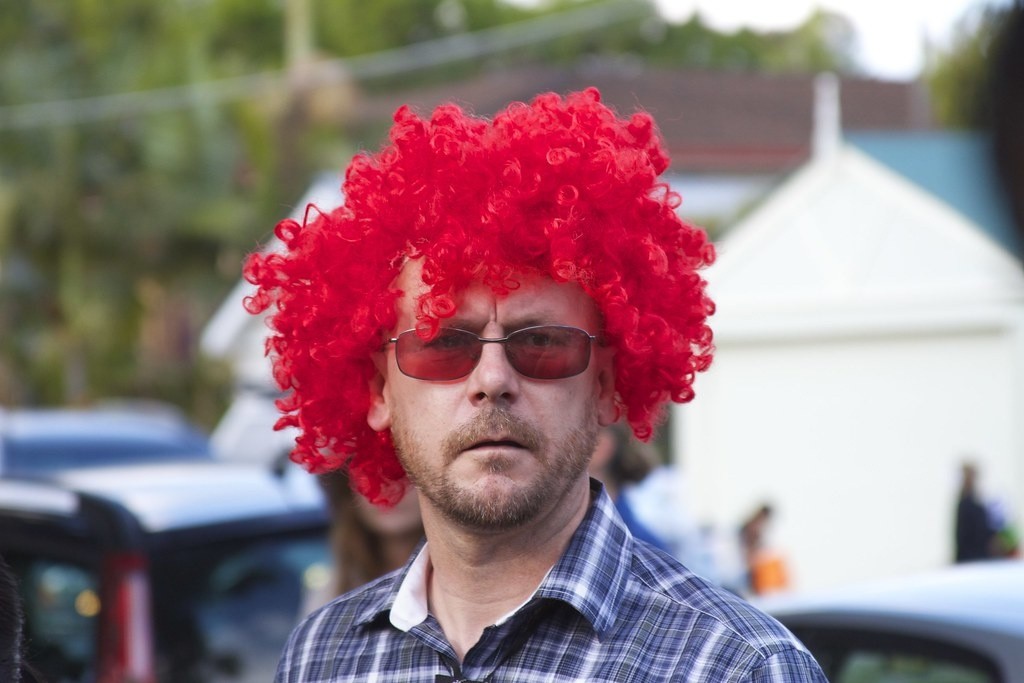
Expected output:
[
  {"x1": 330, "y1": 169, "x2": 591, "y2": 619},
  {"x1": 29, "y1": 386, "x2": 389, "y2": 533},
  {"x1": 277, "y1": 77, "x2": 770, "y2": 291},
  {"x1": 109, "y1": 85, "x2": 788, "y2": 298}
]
[{"x1": 384, "y1": 324, "x2": 604, "y2": 382}]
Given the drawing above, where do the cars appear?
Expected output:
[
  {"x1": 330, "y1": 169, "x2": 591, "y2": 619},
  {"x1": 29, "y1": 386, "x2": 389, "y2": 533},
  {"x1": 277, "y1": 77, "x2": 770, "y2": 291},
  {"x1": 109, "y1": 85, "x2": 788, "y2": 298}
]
[{"x1": 1, "y1": 400, "x2": 1023, "y2": 683}]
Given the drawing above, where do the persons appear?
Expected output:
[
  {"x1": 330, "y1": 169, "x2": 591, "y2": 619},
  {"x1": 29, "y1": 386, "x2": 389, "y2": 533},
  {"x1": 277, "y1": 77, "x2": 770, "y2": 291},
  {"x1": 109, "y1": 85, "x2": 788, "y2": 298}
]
[
  {"x1": 952, "y1": 456, "x2": 1020, "y2": 562},
  {"x1": 589, "y1": 421, "x2": 676, "y2": 556},
  {"x1": 241, "y1": 87, "x2": 831, "y2": 683},
  {"x1": 735, "y1": 499, "x2": 778, "y2": 594},
  {"x1": 314, "y1": 458, "x2": 428, "y2": 596}
]
